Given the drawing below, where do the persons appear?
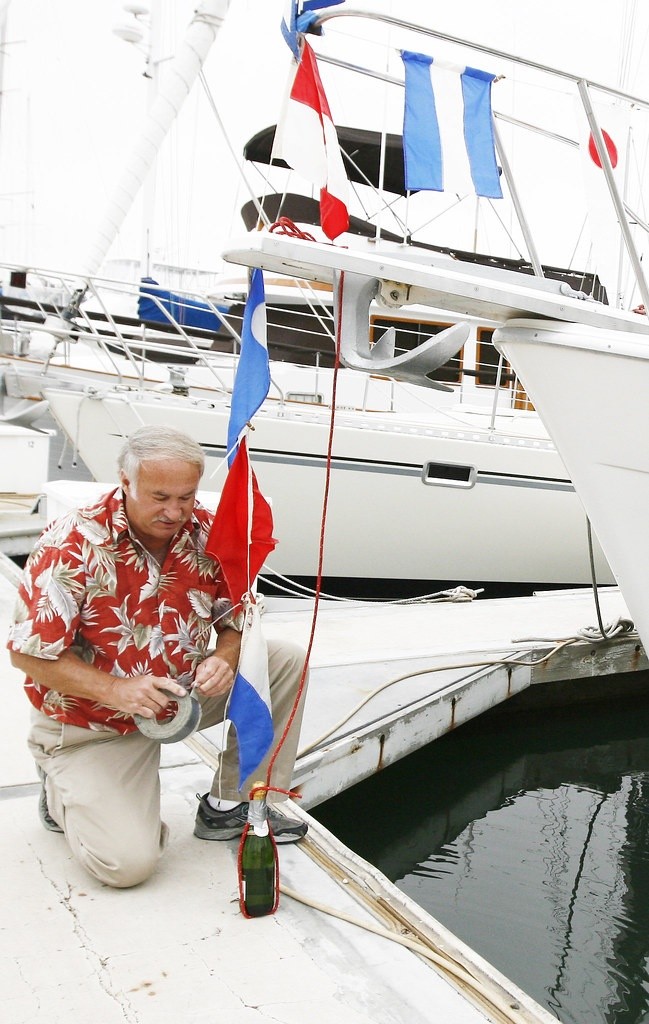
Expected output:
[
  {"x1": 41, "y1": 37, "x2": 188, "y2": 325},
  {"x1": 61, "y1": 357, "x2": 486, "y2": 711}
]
[{"x1": 6, "y1": 423, "x2": 308, "y2": 887}]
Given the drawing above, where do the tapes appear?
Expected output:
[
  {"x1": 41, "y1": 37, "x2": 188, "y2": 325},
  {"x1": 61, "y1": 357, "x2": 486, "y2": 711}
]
[{"x1": 131, "y1": 683, "x2": 202, "y2": 744}]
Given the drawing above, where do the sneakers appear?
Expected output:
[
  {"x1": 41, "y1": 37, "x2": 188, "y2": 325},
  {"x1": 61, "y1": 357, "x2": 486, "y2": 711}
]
[
  {"x1": 193, "y1": 791, "x2": 308, "y2": 845},
  {"x1": 38, "y1": 787, "x2": 63, "y2": 833}
]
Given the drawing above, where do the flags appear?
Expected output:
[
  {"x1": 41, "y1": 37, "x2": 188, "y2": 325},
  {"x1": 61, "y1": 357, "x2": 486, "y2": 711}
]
[
  {"x1": 205, "y1": 436, "x2": 278, "y2": 615},
  {"x1": 272, "y1": 39, "x2": 350, "y2": 241},
  {"x1": 580, "y1": 102, "x2": 628, "y2": 178},
  {"x1": 400, "y1": 49, "x2": 442, "y2": 191},
  {"x1": 227, "y1": 268, "x2": 271, "y2": 468},
  {"x1": 461, "y1": 67, "x2": 503, "y2": 198},
  {"x1": 227, "y1": 606, "x2": 273, "y2": 789}
]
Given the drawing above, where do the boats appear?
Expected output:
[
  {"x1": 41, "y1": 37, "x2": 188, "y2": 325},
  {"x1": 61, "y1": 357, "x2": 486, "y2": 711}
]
[{"x1": 0, "y1": 0, "x2": 649, "y2": 604}]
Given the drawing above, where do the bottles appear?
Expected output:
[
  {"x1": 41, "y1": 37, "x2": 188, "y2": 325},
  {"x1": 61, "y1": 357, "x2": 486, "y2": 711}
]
[{"x1": 242, "y1": 780, "x2": 274, "y2": 917}]
